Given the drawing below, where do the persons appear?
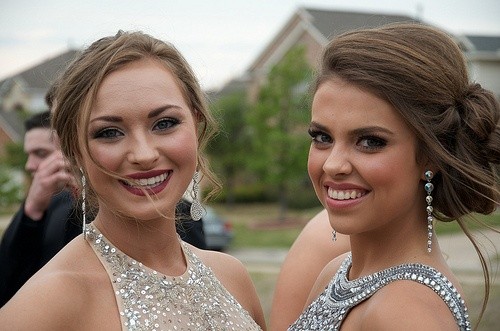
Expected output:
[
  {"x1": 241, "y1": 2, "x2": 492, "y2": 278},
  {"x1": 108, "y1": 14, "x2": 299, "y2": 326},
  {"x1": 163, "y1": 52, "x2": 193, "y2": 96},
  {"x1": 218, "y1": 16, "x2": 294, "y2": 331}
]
[
  {"x1": 0, "y1": 21, "x2": 500, "y2": 331},
  {"x1": 0, "y1": 31, "x2": 267, "y2": 331}
]
